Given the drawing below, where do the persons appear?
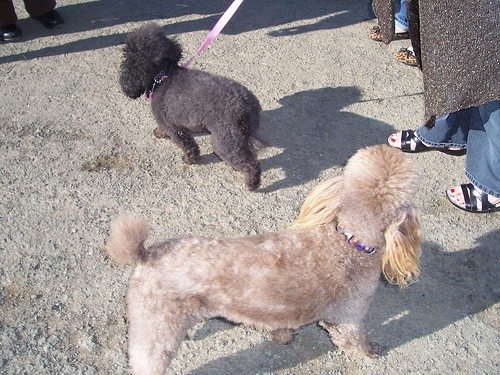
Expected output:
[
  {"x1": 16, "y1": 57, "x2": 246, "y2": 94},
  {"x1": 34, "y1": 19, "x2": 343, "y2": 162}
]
[
  {"x1": 366, "y1": 0, "x2": 500, "y2": 214},
  {"x1": 0, "y1": 0, "x2": 63, "y2": 44}
]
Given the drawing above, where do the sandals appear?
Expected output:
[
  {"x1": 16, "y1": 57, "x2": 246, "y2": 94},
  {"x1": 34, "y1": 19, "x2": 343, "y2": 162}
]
[
  {"x1": 446, "y1": 183, "x2": 500, "y2": 213},
  {"x1": 387, "y1": 130, "x2": 466, "y2": 156}
]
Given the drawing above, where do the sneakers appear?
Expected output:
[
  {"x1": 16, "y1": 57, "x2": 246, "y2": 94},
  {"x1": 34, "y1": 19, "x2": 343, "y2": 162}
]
[
  {"x1": 30, "y1": 9, "x2": 64, "y2": 28},
  {"x1": 0, "y1": 24, "x2": 22, "y2": 40}
]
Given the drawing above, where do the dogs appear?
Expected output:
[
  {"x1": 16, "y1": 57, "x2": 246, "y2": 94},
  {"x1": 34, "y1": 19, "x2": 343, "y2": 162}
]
[
  {"x1": 106, "y1": 144, "x2": 422, "y2": 375},
  {"x1": 119, "y1": 20, "x2": 262, "y2": 190}
]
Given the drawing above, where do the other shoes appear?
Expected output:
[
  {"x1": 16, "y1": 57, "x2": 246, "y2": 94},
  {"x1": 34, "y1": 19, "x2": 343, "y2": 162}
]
[
  {"x1": 368, "y1": 26, "x2": 411, "y2": 41},
  {"x1": 393, "y1": 47, "x2": 417, "y2": 66}
]
